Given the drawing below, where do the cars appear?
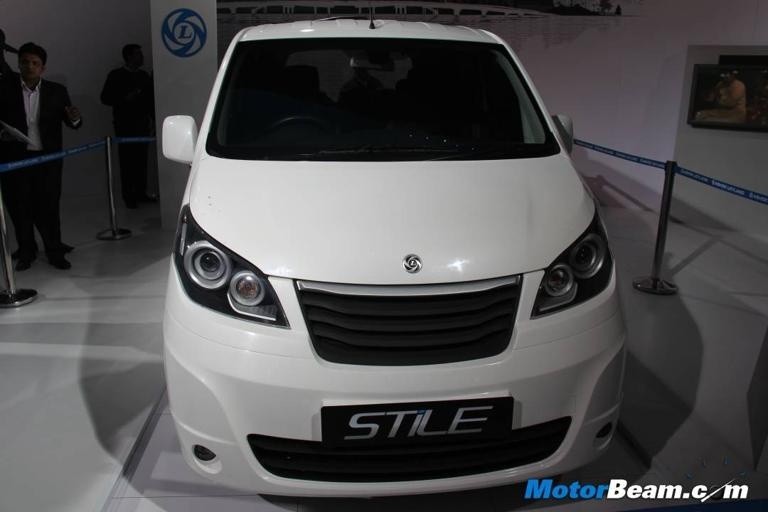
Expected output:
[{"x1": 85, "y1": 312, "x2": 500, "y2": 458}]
[{"x1": 152, "y1": 8, "x2": 634, "y2": 501}]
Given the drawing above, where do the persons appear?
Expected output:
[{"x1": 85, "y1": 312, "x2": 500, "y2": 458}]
[
  {"x1": 0, "y1": 41, "x2": 79, "y2": 270},
  {"x1": 99, "y1": 44, "x2": 156, "y2": 210},
  {"x1": 695, "y1": 68, "x2": 747, "y2": 123}
]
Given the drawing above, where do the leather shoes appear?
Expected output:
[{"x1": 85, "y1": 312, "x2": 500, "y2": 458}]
[{"x1": 11, "y1": 243, "x2": 74, "y2": 271}]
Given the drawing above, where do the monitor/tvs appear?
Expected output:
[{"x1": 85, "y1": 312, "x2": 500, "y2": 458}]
[{"x1": 687, "y1": 63, "x2": 768, "y2": 133}]
